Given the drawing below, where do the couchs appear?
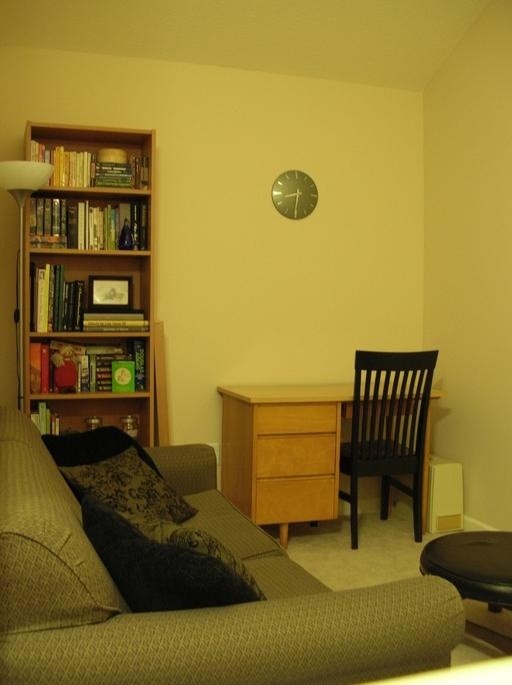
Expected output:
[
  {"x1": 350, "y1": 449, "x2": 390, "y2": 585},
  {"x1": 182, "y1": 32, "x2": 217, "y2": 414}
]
[{"x1": 0, "y1": 405, "x2": 467, "y2": 685}]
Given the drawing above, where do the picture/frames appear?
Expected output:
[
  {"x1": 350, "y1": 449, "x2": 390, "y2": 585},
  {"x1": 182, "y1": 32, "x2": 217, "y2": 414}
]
[{"x1": 83, "y1": 274, "x2": 135, "y2": 315}]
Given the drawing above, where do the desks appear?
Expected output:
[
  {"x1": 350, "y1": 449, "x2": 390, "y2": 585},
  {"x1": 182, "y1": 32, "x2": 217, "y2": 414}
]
[{"x1": 214, "y1": 384, "x2": 441, "y2": 550}]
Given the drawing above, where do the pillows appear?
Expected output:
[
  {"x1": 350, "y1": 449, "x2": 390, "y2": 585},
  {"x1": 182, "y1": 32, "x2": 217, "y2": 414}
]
[
  {"x1": 105, "y1": 507, "x2": 267, "y2": 602},
  {"x1": 58, "y1": 448, "x2": 200, "y2": 525},
  {"x1": 81, "y1": 504, "x2": 261, "y2": 614},
  {"x1": 42, "y1": 424, "x2": 162, "y2": 492}
]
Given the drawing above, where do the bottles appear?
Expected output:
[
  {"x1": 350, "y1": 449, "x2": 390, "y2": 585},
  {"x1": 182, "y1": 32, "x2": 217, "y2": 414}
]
[
  {"x1": 85, "y1": 415, "x2": 103, "y2": 431},
  {"x1": 120, "y1": 415, "x2": 138, "y2": 441}
]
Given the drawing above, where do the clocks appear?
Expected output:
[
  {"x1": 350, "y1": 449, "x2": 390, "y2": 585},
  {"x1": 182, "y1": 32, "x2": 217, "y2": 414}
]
[{"x1": 270, "y1": 169, "x2": 317, "y2": 218}]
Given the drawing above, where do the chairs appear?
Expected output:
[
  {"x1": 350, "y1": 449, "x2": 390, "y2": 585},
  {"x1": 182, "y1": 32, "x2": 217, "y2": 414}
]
[{"x1": 310, "y1": 347, "x2": 440, "y2": 551}]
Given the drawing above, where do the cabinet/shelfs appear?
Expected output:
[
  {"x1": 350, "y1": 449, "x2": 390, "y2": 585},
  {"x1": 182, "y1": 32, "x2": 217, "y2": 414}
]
[{"x1": 23, "y1": 118, "x2": 158, "y2": 447}]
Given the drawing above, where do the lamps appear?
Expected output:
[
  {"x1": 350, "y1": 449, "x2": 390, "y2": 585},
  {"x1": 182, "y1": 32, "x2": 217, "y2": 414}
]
[{"x1": 0, "y1": 159, "x2": 56, "y2": 409}]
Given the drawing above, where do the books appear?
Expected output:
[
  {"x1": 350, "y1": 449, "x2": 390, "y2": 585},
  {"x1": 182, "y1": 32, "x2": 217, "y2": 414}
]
[{"x1": 31, "y1": 139, "x2": 150, "y2": 435}]
